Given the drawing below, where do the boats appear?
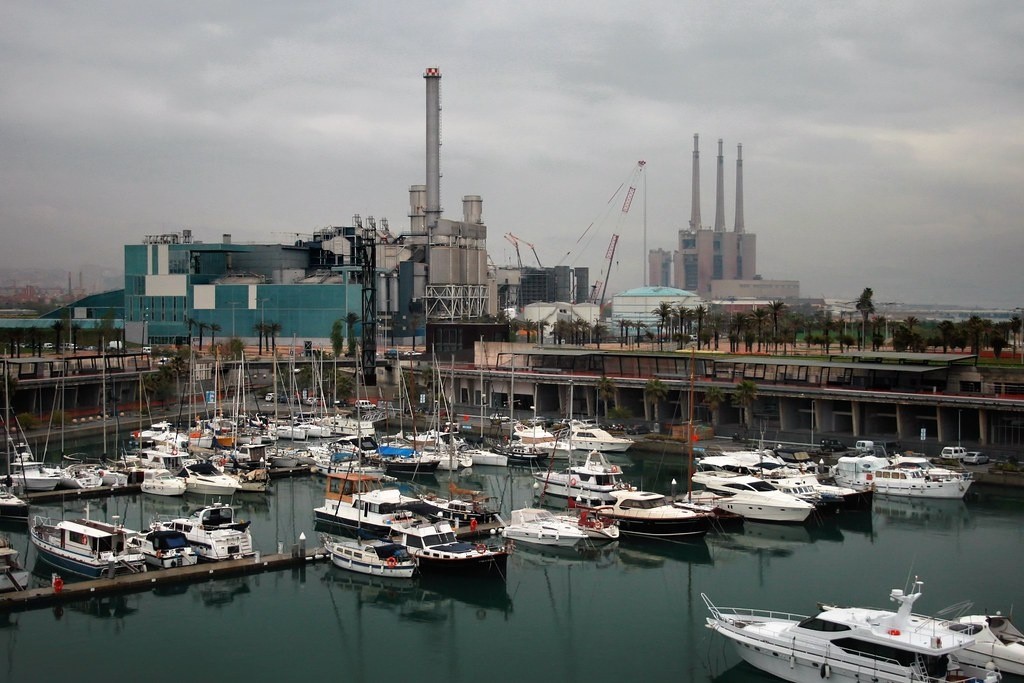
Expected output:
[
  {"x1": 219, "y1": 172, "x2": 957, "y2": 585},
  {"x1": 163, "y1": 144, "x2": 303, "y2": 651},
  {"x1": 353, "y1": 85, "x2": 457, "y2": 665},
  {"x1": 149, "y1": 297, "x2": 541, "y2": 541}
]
[
  {"x1": 168, "y1": 495, "x2": 256, "y2": 561},
  {"x1": 121, "y1": 520, "x2": 200, "y2": 569},
  {"x1": 814, "y1": 597, "x2": 1024, "y2": 678},
  {"x1": 699, "y1": 573, "x2": 1004, "y2": 683},
  {"x1": 29, "y1": 501, "x2": 147, "y2": 581}
]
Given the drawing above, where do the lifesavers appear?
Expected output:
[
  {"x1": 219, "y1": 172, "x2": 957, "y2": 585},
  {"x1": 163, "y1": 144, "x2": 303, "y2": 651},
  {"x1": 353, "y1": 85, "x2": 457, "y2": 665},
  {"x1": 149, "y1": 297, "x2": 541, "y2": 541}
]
[
  {"x1": 593, "y1": 521, "x2": 602, "y2": 531},
  {"x1": 570, "y1": 478, "x2": 576, "y2": 485},
  {"x1": 470, "y1": 520, "x2": 477, "y2": 528},
  {"x1": 477, "y1": 544, "x2": 487, "y2": 553},
  {"x1": 82, "y1": 535, "x2": 87, "y2": 545},
  {"x1": 156, "y1": 551, "x2": 163, "y2": 559},
  {"x1": 887, "y1": 630, "x2": 901, "y2": 635},
  {"x1": 54, "y1": 578, "x2": 63, "y2": 589},
  {"x1": 611, "y1": 465, "x2": 617, "y2": 472},
  {"x1": 387, "y1": 557, "x2": 396, "y2": 568}
]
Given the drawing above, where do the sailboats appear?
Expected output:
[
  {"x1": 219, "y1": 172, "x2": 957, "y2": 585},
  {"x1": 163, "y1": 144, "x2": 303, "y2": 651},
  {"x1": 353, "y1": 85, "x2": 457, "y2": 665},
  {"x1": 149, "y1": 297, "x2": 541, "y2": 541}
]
[{"x1": 0, "y1": 348, "x2": 977, "y2": 578}]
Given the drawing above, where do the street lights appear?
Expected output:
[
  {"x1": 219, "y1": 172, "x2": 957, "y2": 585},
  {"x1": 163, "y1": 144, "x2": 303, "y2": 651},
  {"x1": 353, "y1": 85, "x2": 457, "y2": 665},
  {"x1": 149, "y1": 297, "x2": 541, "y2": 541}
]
[
  {"x1": 811, "y1": 400, "x2": 817, "y2": 451},
  {"x1": 959, "y1": 409, "x2": 963, "y2": 464}
]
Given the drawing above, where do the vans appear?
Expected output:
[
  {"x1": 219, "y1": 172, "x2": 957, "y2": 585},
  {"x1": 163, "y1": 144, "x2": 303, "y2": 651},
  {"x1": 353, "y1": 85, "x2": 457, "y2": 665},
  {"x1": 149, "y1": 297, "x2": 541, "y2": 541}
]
[{"x1": 939, "y1": 447, "x2": 968, "y2": 462}]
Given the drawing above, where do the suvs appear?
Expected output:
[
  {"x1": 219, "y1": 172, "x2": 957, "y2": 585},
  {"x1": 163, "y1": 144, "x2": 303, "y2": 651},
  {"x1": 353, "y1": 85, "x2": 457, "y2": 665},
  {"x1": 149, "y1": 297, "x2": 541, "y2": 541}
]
[
  {"x1": 353, "y1": 399, "x2": 376, "y2": 410},
  {"x1": 856, "y1": 440, "x2": 904, "y2": 458},
  {"x1": 820, "y1": 438, "x2": 847, "y2": 452}
]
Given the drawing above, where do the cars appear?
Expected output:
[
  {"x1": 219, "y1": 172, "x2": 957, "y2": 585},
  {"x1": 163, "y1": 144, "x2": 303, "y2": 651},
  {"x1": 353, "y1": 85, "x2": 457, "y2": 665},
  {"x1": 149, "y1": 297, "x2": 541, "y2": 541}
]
[
  {"x1": 489, "y1": 412, "x2": 510, "y2": 422},
  {"x1": 963, "y1": 451, "x2": 990, "y2": 465},
  {"x1": 529, "y1": 416, "x2": 623, "y2": 430},
  {"x1": 996, "y1": 454, "x2": 1017, "y2": 468},
  {"x1": 158, "y1": 357, "x2": 170, "y2": 365},
  {"x1": 99, "y1": 408, "x2": 118, "y2": 418},
  {"x1": 264, "y1": 392, "x2": 348, "y2": 408},
  {"x1": 626, "y1": 424, "x2": 650, "y2": 435},
  {"x1": 140, "y1": 346, "x2": 152, "y2": 354},
  {"x1": 405, "y1": 403, "x2": 458, "y2": 418}
]
